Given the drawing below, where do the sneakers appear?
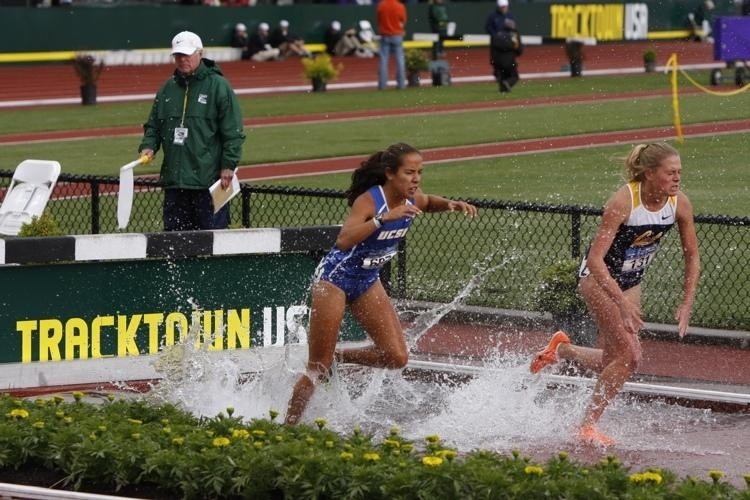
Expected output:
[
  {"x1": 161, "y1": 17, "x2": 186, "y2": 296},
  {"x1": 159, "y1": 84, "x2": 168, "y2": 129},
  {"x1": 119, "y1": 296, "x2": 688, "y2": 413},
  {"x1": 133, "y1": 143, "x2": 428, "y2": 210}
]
[
  {"x1": 578, "y1": 425, "x2": 613, "y2": 447},
  {"x1": 530, "y1": 331, "x2": 569, "y2": 373}
]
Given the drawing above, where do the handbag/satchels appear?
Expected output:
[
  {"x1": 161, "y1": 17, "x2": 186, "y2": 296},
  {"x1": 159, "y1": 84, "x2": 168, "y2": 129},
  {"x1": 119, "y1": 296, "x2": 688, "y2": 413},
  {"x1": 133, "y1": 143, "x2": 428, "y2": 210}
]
[{"x1": 503, "y1": 30, "x2": 522, "y2": 51}]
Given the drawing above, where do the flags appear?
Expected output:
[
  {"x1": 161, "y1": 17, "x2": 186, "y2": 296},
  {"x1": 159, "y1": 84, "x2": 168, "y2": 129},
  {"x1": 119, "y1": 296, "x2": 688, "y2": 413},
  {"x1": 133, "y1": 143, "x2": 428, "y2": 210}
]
[{"x1": 117, "y1": 169, "x2": 134, "y2": 228}]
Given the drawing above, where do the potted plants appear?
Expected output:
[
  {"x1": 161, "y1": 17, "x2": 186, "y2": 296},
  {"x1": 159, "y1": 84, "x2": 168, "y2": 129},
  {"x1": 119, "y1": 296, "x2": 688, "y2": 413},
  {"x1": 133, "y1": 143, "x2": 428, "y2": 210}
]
[
  {"x1": 563, "y1": 40, "x2": 584, "y2": 76},
  {"x1": 300, "y1": 52, "x2": 343, "y2": 91},
  {"x1": 403, "y1": 47, "x2": 431, "y2": 87},
  {"x1": 641, "y1": 48, "x2": 658, "y2": 72},
  {"x1": 70, "y1": 53, "x2": 106, "y2": 106}
]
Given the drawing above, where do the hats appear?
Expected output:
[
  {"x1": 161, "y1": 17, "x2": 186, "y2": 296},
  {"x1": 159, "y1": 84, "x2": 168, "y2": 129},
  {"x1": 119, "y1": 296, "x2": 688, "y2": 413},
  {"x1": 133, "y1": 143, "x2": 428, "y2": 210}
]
[
  {"x1": 235, "y1": 19, "x2": 289, "y2": 30},
  {"x1": 171, "y1": 31, "x2": 204, "y2": 56},
  {"x1": 332, "y1": 20, "x2": 342, "y2": 32},
  {"x1": 497, "y1": 0, "x2": 509, "y2": 7}
]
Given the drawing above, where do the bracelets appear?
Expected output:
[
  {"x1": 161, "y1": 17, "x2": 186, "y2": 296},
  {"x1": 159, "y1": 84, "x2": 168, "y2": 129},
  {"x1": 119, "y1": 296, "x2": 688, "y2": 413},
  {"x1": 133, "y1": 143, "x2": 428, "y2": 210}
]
[{"x1": 373, "y1": 217, "x2": 382, "y2": 229}]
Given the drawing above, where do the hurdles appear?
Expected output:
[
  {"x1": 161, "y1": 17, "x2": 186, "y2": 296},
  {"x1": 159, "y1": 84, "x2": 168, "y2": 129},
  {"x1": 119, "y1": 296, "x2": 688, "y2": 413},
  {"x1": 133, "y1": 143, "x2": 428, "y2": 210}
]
[{"x1": 0, "y1": 224, "x2": 391, "y2": 398}]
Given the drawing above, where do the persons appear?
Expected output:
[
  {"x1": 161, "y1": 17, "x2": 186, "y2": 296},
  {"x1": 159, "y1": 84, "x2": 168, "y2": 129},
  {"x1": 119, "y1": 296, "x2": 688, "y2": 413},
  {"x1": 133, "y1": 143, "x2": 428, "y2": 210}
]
[
  {"x1": 489, "y1": 18, "x2": 523, "y2": 93},
  {"x1": 231, "y1": 19, "x2": 383, "y2": 63},
  {"x1": 528, "y1": 142, "x2": 700, "y2": 449},
  {"x1": 138, "y1": 30, "x2": 247, "y2": 232},
  {"x1": 428, "y1": 0, "x2": 449, "y2": 60},
  {"x1": 373, "y1": 0, "x2": 408, "y2": 90},
  {"x1": 486, "y1": 0, "x2": 518, "y2": 81},
  {"x1": 284, "y1": 142, "x2": 478, "y2": 426}
]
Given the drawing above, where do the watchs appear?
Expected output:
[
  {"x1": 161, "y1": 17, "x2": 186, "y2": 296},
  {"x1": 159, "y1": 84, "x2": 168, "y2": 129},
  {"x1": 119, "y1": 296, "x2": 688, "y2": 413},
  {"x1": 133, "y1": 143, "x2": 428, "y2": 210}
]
[{"x1": 375, "y1": 212, "x2": 385, "y2": 227}]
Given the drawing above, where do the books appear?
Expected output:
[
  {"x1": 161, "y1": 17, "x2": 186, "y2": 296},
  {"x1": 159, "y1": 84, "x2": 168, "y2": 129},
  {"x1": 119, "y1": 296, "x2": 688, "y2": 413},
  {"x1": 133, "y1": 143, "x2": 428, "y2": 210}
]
[{"x1": 209, "y1": 172, "x2": 241, "y2": 216}]
